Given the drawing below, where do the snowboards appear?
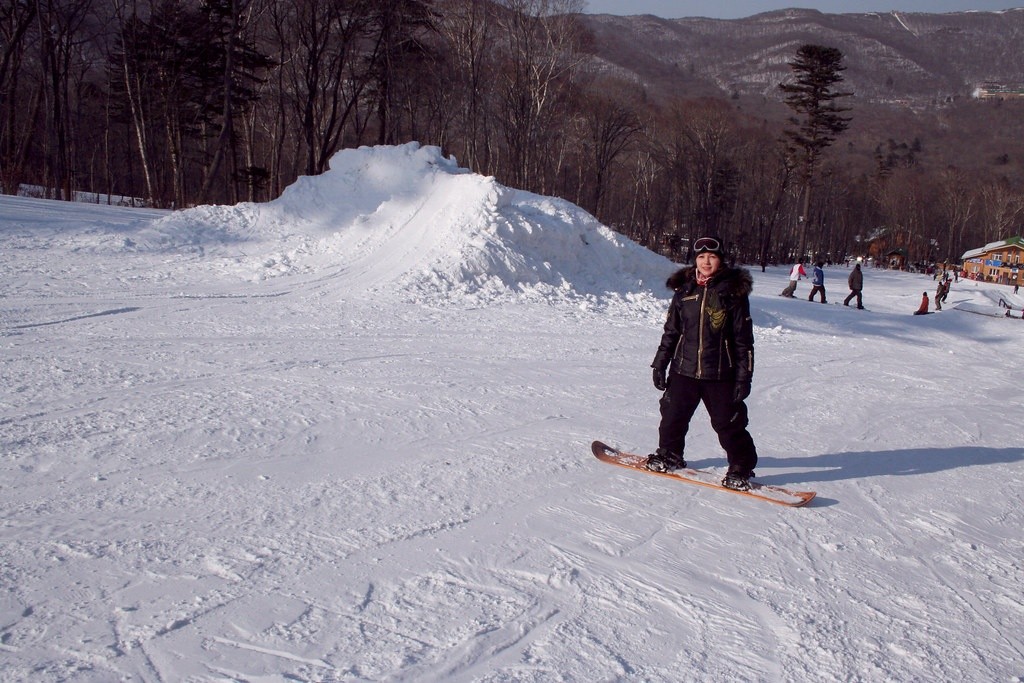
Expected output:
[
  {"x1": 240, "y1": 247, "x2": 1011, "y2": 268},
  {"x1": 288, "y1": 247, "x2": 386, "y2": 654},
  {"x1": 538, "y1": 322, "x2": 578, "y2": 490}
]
[{"x1": 588, "y1": 436, "x2": 821, "y2": 512}]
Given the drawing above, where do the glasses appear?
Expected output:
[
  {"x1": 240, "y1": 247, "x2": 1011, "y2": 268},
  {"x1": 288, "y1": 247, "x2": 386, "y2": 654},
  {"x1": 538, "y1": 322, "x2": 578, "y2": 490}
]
[{"x1": 694, "y1": 237, "x2": 719, "y2": 251}]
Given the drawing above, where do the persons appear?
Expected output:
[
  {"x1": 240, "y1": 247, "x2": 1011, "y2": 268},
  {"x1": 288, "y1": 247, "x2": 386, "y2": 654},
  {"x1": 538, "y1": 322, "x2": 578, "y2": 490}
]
[
  {"x1": 761, "y1": 258, "x2": 767, "y2": 274},
  {"x1": 646, "y1": 227, "x2": 757, "y2": 490},
  {"x1": 782, "y1": 257, "x2": 808, "y2": 298},
  {"x1": 844, "y1": 263, "x2": 864, "y2": 309},
  {"x1": 809, "y1": 261, "x2": 827, "y2": 303},
  {"x1": 872, "y1": 259, "x2": 1019, "y2": 314}
]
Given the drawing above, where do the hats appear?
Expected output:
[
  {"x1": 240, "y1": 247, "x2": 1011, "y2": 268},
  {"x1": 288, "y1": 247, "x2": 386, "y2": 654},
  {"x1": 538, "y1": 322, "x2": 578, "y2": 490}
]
[{"x1": 693, "y1": 237, "x2": 724, "y2": 262}]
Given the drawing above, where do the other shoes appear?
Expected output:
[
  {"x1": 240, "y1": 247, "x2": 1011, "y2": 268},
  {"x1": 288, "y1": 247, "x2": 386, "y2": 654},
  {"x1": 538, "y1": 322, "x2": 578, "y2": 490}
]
[
  {"x1": 722, "y1": 470, "x2": 754, "y2": 491},
  {"x1": 858, "y1": 306, "x2": 864, "y2": 309},
  {"x1": 823, "y1": 301, "x2": 827, "y2": 303},
  {"x1": 646, "y1": 447, "x2": 687, "y2": 473}
]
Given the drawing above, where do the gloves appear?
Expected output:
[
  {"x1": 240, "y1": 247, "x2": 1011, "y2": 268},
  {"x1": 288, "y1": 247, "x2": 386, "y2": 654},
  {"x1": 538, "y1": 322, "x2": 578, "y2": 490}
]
[
  {"x1": 652, "y1": 369, "x2": 668, "y2": 390},
  {"x1": 731, "y1": 382, "x2": 751, "y2": 404}
]
